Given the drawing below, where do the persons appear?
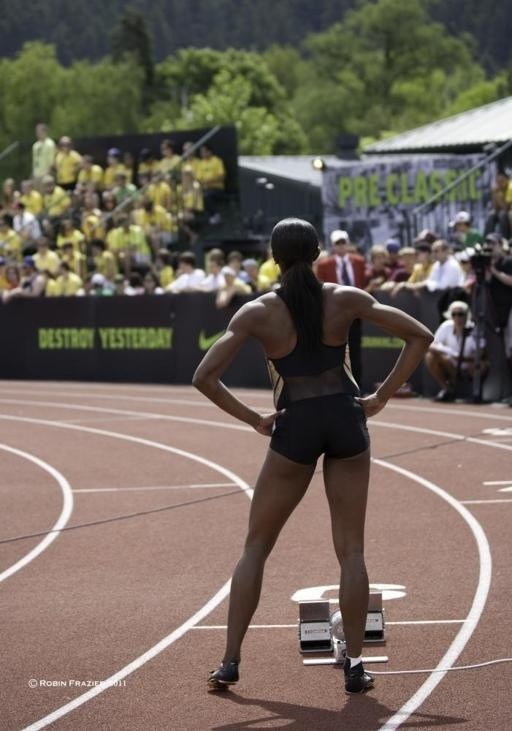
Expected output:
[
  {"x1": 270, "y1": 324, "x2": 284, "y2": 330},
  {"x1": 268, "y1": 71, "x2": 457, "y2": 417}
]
[
  {"x1": 318, "y1": 231, "x2": 366, "y2": 384},
  {"x1": 147, "y1": 247, "x2": 281, "y2": 308},
  {"x1": 369, "y1": 169, "x2": 512, "y2": 300},
  {"x1": 0, "y1": 122, "x2": 226, "y2": 305},
  {"x1": 423, "y1": 301, "x2": 486, "y2": 403},
  {"x1": 191, "y1": 216, "x2": 433, "y2": 698},
  {"x1": 464, "y1": 232, "x2": 511, "y2": 404}
]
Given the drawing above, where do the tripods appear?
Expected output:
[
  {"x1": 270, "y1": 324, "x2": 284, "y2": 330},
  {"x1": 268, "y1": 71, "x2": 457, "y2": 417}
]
[{"x1": 454, "y1": 272, "x2": 512, "y2": 405}]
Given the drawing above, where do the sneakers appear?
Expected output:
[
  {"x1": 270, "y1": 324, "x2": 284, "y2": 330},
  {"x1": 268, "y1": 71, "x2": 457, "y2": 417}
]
[
  {"x1": 207, "y1": 660, "x2": 239, "y2": 684},
  {"x1": 344, "y1": 657, "x2": 375, "y2": 695}
]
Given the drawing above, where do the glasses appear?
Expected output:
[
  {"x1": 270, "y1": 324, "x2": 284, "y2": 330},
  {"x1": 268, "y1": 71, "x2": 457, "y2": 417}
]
[{"x1": 452, "y1": 311, "x2": 465, "y2": 316}]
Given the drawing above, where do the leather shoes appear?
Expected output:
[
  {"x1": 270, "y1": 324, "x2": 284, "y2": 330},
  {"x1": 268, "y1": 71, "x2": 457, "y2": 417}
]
[{"x1": 434, "y1": 391, "x2": 454, "y2": 401}]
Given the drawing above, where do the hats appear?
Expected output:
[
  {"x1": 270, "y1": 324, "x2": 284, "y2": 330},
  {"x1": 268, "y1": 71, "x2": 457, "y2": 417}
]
[
  {"x1": 485, "y1": 234, "x2": 503, "y2": 245},
  {"x1": 331, "y1": 229, "x2": 349, "y2": 242},
  {"x1": 448, "y1": 210, "x2": 471, "y2": 227}
]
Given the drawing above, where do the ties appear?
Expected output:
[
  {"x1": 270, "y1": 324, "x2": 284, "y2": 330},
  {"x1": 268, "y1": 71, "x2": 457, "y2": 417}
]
[{"x1": 341, "y1": 261, "x2": 352, "y2": 285}]
